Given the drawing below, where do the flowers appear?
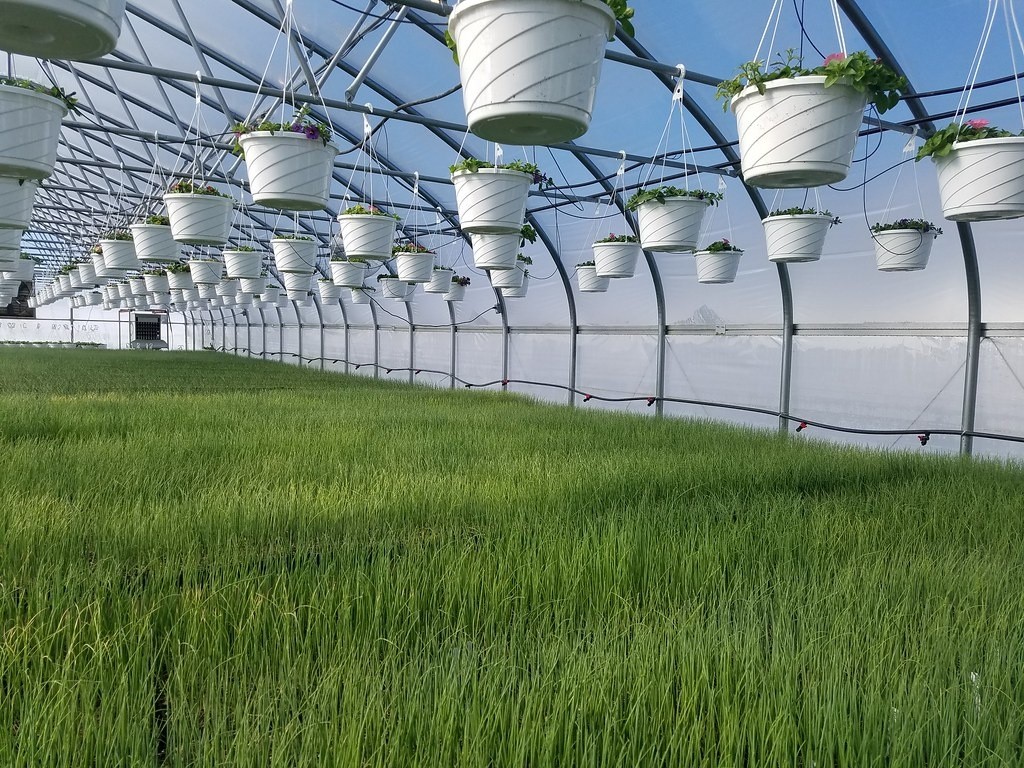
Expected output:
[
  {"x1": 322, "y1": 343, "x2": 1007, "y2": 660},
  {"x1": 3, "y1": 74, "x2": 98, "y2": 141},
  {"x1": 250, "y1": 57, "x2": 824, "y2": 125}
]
[{"x1": 93, "y1": 0, "x2": 1024, "y2": 256}]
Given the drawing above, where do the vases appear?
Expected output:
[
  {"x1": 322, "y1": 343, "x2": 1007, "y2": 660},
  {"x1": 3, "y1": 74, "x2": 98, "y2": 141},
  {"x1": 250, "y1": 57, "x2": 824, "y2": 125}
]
[{"x1": 0, "y1": 0, "x2": 1024, "y2": 313}]
